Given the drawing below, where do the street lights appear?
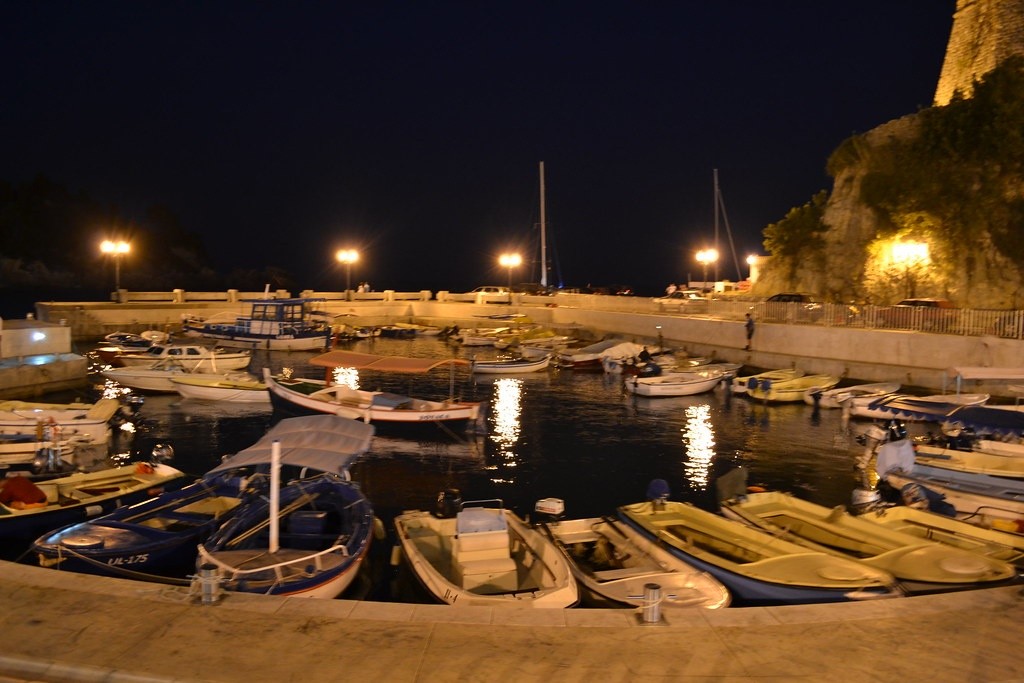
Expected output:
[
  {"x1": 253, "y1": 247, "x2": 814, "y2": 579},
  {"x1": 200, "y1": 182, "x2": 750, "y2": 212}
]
[
  {"x1": 498, "y1": 253, "x2": 521, "y2": 293},
  {"x1": 100, "y1": 240, "x2": 130, "y2": 288},
  {"x1": 335, "y1": 248, "x2": 358, "y2": 300},
  {"x1": 696, "y1": 249, "x2": 719, "y2": 290}
]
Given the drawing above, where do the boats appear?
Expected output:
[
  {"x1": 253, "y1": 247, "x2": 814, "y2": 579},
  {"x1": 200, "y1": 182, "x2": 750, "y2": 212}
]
[
  {"x1": 391, "y1": 485, "x2": 588, "y2": 612},
  {"x1": 717, "y1": 487, "x2": 1024, "y2": 600},
  {"x1": 0, "y1": 386, "x2": 185, "y2": 542},
  {"x1": 623, "y1": 370, "x2": 725, "y2": 398},
  {"x1": 444, "y1": 318, "x2": 746, "y2": 382},
  {"x1": 88, "y1": 289, "x2": 446, "y2": 404},
  {"x1": 729, "y1": 363, "x2": 842, "y2": 403},
  {"x1": 261, "y1": 369, "x2": 485, "y2": 436},
  {"x1": 807, "y1": 374, "x2": 1024, "y2": 577},
  {"x1": 526, "y1": 497, "x2": 733, "y2": 610},
  {"x1": 194, "y1": 476, "x2": 376, "y2": 601},
  {"x1": 617, "y1": 480, "x2": 912, "y2": 604},
  {"x1": 28, "y1": 458, "x2": 285, "y2": 576}
]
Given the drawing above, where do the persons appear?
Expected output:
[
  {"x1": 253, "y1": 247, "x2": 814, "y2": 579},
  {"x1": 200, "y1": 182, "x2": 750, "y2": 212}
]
[
  {"x1": 638, "y1": 346, "x2": 649, "y2": 362},
  {"x1": 666, "y1": 283, "x2": 676, "y2": 295},
  {"x1": 358, "y1": 281, "x2": 370, "y2": 292},
  {"x1": 744, "y1": 313, "x2": 753, "y2": 350}
]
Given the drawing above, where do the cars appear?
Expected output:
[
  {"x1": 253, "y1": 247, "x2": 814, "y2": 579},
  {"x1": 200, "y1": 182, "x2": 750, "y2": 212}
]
[
  {"x1": 748, "y1": 292, "x2": 959, "y2": 334},
  {"x1": 653, "y1": 291, "x2": 711, "y2": 317},
  {"x1": 469, "y1": 282, "x2": 557, "y2": 306}
]
